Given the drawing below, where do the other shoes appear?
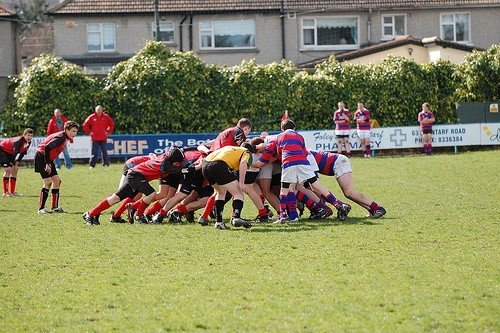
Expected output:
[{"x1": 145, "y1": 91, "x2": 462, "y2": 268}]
[
  {"x1": 424, "y1": 153, "x2": 432, "y2": 156},
  {"x1": 364, "y1": 153, "x2": 371, "y2": 158},
  {"x1": 89, "y1": 166, "x2": 93, "y2": 169}
]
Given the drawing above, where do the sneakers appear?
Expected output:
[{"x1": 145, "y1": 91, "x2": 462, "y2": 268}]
[
  {"x1": 92, "y1": 215, "x2": 101, "y2": 225},
  {"x1": 38, "y1": 208, "x2": 48, "y2": 214},
  {"x1": 230, "y1": 218, "x2": 252, "y2": 229},
  {"x1": 215, "y1": 222, "x2": 230, "y2": 230},
  {"x1": 264, "y1": 205, "x2": 274, "y2": 218},
  {"x1": 152, "y1": 213, "x2": 164, "y2": 224},
  {"x1": 10, "y1": 192, "x2": 18, "y2": 196},
  {"x1": 308, "y1": 205, "x2": 332, "y2": 220},
  {"x1": 135, "y1": 215, "x2": 147, "y2": 224},
  {"x1": 3, "y1": 192, "x2": 12, "y2": 197},
  {"x1": 365, "y1": 211, "x2": 376, "y2": 219},
  {"x1": 198, "y1": 216, "x2": 214, "y2": 226},
  {"x1": 110, "y1": 212, "x2": 126, "y2": 223},
  {"x1": 251, "y1": 215, "x2": 270, "y2": 223},
  {"x1": 143, "y1": 212, "x2": 152, "y2": 223},
  {"x1": 84, "y1": 211, "x2": 94, "y2": 226},
  {"x1": 297, "y1": 201, "x2": 304, "y2": 211},
  {"x1": 210, "y1": 205, "x2": 217, "y2": 220},
  {"x1": 50, "y1": 207, "x2": 63, "y2": 213},
  {"x1": 335, "y1": 202, "x2": 351, "y2": 221},
  {"x1": 371, "y1": 207, "x2": 386, "y2": 219},
  {"x1": 126, "y1": 203, "x2": 137, "y2": 225},
  {"x1": 273, "y1": 210, "x2": 299, "y2": 223},
  {"x1": 166, "y1": 208, "x2": 196, "y2": 224}
]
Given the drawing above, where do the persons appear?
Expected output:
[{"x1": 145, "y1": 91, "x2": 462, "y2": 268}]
[
  {"x1": 34, "y1": 121, "x2": 79, "y2": 214},
  {"x1": 47, "y1": 109, "x2": 72, "y2": 170},
  {"x1": 0, "y1": 128, "x2": 33, "y2": 197},
  {"x1": 82, "y1": 105, "x2": 114, "y2": 169},
  {"x1": 354, "y1": 102, "x2": 370, "y2": 158},
  {"x1": 418, "y1": 103, "x2": 435, "y2": 155},
  {"x1": 333, "y1": 102, "x2": 352, "y2": 158},
  {"x1": 82, "y1": 118, "x2": 387, "y2": 229}
]
